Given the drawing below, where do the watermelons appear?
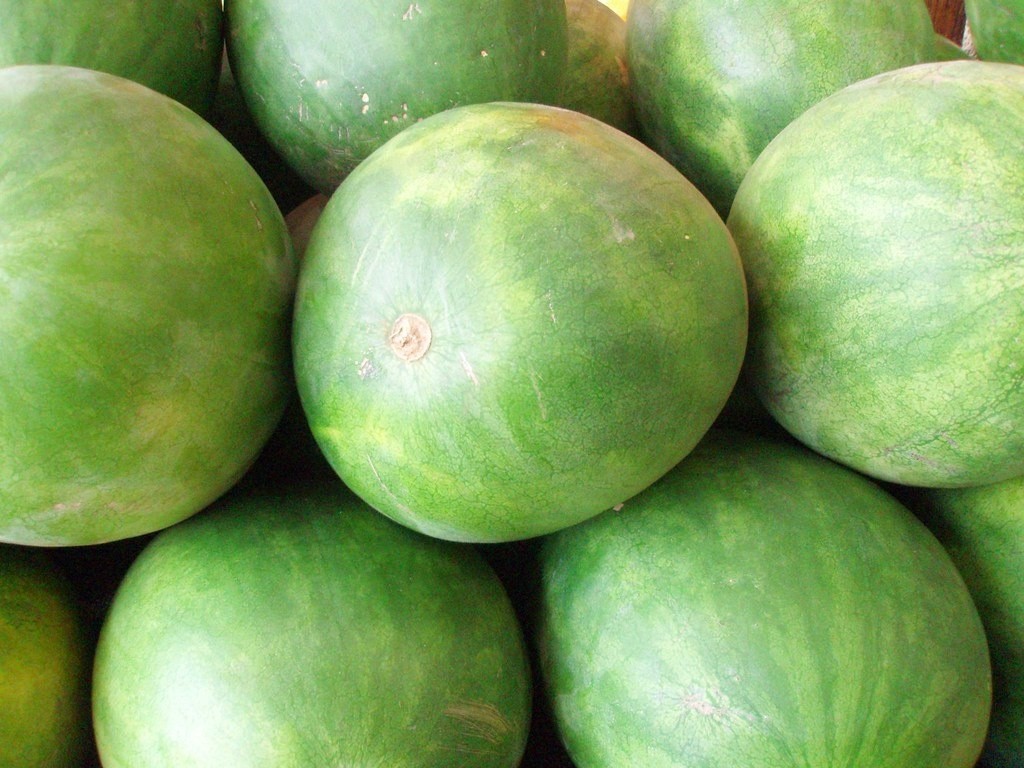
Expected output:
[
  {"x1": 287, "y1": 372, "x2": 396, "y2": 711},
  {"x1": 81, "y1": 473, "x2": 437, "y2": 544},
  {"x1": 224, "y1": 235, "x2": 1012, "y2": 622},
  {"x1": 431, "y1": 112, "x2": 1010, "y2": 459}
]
[{"x1": 0, "y1": 0, "x2": 1024, "y2": 768}]
